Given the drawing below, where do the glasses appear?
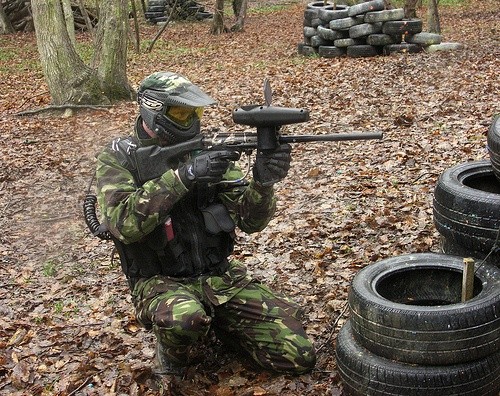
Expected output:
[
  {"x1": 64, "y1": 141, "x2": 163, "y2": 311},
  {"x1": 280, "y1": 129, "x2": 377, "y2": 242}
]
[{"x1": 166, "y1": 105, "x2": 204, "y2": 128}]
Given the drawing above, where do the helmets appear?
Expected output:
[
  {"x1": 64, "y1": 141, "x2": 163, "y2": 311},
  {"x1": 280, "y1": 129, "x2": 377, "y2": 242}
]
[{"x1": 136, "y1": 71, "x2": 218, "y2": 148}]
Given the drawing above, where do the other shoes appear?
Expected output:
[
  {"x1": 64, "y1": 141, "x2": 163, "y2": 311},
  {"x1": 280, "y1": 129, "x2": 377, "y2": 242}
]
[{"x1": 157, "y1": 339, "x2": 191, "y2": 377}]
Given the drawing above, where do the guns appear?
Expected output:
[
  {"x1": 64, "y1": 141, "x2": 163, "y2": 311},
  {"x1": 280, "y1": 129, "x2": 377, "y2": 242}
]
[{"x1": 134, "y1": 78, "x2": 384, "y2": 183}]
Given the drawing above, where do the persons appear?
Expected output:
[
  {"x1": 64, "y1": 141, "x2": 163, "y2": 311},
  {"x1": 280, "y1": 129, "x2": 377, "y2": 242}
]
[{"x1": 94, "y1": 69, "x2": 317, "y2": 382}]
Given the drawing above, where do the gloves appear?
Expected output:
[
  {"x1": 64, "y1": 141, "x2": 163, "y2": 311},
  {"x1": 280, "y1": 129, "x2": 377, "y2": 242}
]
[
  {"x1": 262, "y1": 142, "x2": 292, "y2": 180},
  {"x1": 177, "y1": 144, "x2": 237, "y2": 193}
]
